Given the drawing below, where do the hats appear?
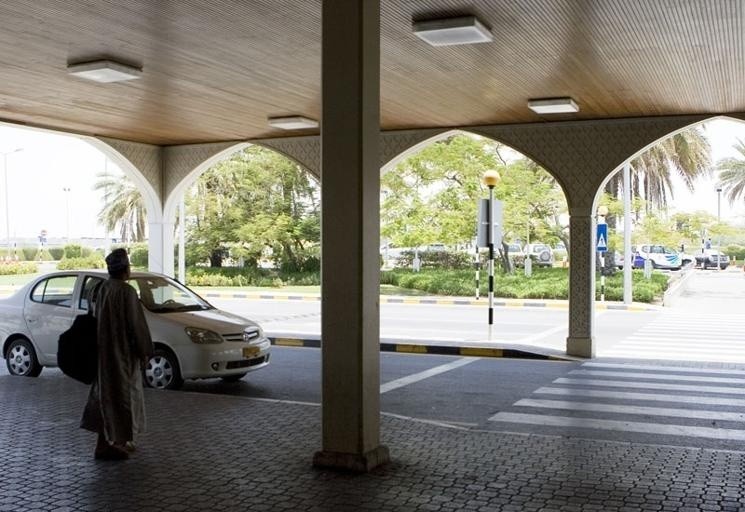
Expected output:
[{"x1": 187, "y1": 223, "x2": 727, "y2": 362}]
[{"x1": 103, "y1": 247, "x2": 131, "y2": 269}]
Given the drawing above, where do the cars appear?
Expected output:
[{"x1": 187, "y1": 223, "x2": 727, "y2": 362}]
[{"x1": 0, "y1": 267, "x2": 273, "y2": 391}]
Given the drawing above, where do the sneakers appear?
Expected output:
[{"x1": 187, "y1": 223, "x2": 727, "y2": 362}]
[
  {"x1": 111, "y1": 440, "x2": 138, "y2": 452},
  {"x1": 94, "y1": 445, "x2": 130, "y2": 461}
]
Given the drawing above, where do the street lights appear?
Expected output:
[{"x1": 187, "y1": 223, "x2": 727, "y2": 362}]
[
  {"x1": 716, "y1": 186, "x2": 722, "y2": 272},
  {"x1": 597, "y1": 204, "x2": 610, "y2": 312},
  {"x1": 481, "y1": 168, "x2": 502, "y2": 344}
]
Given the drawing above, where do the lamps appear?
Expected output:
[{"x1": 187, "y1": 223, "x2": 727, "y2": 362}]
[
  {"x1": 267, "y1": 115, "x2": 319, "y2": 130},
  {"x1": 526, "y1": 96, "x2": 580, "y2": 115},
  {"x1": 66, "y1": 59, "x2": 150, "y2": 81},
  {"x1": 411, "y1": 13, "x2": 494, "y2": 48}
]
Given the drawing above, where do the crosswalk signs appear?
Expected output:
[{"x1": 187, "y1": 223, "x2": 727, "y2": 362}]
[{"x1": 598, "y1": 224, "x2": 607, "y2": 251}]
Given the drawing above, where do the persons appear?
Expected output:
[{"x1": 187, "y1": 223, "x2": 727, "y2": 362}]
[
  {"x1": 705, "y1": 238, "x2": 712, "y2": 249},
  {"x1": 77, "y1": 247, "x2": 154, "y2": 461}
]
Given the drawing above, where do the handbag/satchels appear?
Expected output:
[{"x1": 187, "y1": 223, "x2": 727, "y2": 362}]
[{"x1": 55, "y1": 313, "x2": 99, "y2": 387}]
